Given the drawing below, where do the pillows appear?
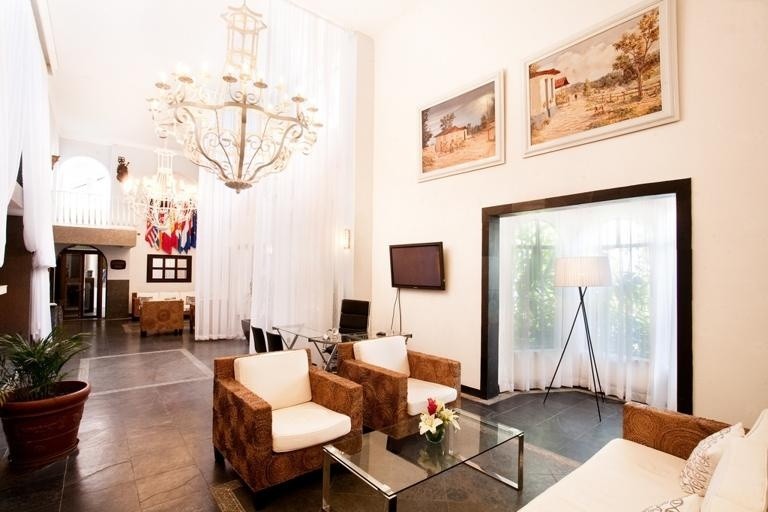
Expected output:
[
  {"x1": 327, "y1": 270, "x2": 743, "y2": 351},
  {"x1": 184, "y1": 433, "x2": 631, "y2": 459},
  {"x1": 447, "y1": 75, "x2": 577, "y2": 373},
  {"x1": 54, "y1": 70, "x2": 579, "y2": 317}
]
[
  {"x1": 641, "y1": 494, "x2": 700, "y2": 512},
  {"x1": 678, "y1": 421, "x2": 746, "y2": 498},
  {"x1": 701, "y1": 407, "x2": 768, "y2": 512}
]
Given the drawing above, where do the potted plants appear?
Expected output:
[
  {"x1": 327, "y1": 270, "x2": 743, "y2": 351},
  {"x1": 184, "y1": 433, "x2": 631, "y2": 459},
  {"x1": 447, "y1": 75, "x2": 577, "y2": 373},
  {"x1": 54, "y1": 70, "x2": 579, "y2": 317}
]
[{"x1": 0, "y1": 324, "x2": 100, "y2": 468}]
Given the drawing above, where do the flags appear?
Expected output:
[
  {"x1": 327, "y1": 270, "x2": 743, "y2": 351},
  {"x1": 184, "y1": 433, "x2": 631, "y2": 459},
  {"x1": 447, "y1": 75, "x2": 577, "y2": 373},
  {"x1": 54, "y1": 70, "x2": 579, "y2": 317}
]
[{"x1": 145, "y1": 196, "x2": 197, "y2": 252}]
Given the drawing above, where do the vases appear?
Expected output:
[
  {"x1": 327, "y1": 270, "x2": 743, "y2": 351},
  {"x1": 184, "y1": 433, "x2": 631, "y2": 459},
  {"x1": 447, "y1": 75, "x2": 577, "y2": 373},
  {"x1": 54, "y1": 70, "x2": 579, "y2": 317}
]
[{"x1": 424, "y1": 425, "x2": 446, "y2": 443}]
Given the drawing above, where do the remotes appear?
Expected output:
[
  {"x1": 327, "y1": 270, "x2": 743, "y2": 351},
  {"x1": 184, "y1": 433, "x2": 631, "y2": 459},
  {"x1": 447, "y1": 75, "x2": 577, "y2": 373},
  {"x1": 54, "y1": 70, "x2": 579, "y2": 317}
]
[{"x1": 376, "y1": 332, "x2": 386, "y2": 335}]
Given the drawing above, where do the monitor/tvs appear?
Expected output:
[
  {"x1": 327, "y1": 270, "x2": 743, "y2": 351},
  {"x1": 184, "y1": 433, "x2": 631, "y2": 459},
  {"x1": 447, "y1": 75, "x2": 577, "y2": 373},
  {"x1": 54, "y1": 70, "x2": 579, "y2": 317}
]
[{"x1": 389, "y1": 241, "x2": 446, "y2": 291}]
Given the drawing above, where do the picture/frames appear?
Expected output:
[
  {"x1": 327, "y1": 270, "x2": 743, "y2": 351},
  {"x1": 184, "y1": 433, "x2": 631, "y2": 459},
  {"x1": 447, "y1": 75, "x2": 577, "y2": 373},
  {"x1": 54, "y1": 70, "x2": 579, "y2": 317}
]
[
  {"x1": 521, "y1": 0, "x2": 682, "y2": 163},
  {"x1": 410, "y1": 69, "x2": 506, "y2": 186}
]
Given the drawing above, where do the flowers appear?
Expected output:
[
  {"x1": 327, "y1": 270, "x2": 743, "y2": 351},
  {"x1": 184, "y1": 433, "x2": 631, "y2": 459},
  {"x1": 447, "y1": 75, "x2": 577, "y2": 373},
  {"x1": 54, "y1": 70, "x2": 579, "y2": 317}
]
[{"x1": 415, "y1": 395, "x2": 462, "y2": 443}]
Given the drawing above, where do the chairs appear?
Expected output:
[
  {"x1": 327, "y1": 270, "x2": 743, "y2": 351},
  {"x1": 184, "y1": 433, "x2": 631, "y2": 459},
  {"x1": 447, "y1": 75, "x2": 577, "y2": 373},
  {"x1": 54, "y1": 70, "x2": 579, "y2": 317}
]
[
  {"x1": 322, "y1": 297, "x2": 370, "y2": 359},
  {"x1": 250, "y1": 325, "x2": 267, "y2": 354},
  {"x1": 264, "y1": 330, "x2": 283, "y2": 353}
]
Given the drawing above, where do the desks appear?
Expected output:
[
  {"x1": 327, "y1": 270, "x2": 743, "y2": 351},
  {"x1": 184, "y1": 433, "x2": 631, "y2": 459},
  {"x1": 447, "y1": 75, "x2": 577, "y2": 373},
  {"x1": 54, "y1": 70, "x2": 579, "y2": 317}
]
[
  {"x1": 271, "y1": 321, "x2": 366, "y2": 372},
  {"x1": 188, "y1": 303, "x2": 196, "y2": 333}
]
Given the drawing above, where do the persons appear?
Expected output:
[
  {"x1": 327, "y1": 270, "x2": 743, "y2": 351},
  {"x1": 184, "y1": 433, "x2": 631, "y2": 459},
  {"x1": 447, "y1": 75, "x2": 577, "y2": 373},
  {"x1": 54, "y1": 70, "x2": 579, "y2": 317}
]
[{"x1": 436, "y1": 131, "x2": 466, "y2": 157}]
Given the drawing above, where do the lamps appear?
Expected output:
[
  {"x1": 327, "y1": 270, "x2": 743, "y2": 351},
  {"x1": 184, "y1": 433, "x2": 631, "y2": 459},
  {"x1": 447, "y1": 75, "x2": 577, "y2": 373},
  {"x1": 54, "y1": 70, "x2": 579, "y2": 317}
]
[
  {"x1": 541, "y1": 254, "x2": 614, "y2": 421},
  {"x1": 120, "y1": 141, "x2": 203, "y2": 235},
  {"x1": 146, "y1": 3, "x2": 325, "y2": 196}
]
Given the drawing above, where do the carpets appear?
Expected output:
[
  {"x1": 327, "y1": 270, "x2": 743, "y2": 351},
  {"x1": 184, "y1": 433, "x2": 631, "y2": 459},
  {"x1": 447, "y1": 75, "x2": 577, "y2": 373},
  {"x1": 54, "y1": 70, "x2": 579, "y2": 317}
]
[{"x1": 208, "y1": 413, "x2": 583, "y2": 512}]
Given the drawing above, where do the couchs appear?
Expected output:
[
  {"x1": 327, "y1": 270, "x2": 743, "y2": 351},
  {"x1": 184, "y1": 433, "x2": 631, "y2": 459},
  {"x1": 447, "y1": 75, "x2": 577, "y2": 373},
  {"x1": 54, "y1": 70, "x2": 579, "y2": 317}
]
[
  {"x1": 132, "y1": 290, "x2": 195, "y2": 320},
  {"x1": 515, "y1": 400, "x2": 768, "y2": 512},
  {"x1": 208, "y1": 346, "x2": 366, "y2": 509},
  {"x1": 335, "y1": 335, "x2": 464, "y2": 454},
  {"x1": 138, "y1": 298, "x2": 184, "y2": 338}
]
